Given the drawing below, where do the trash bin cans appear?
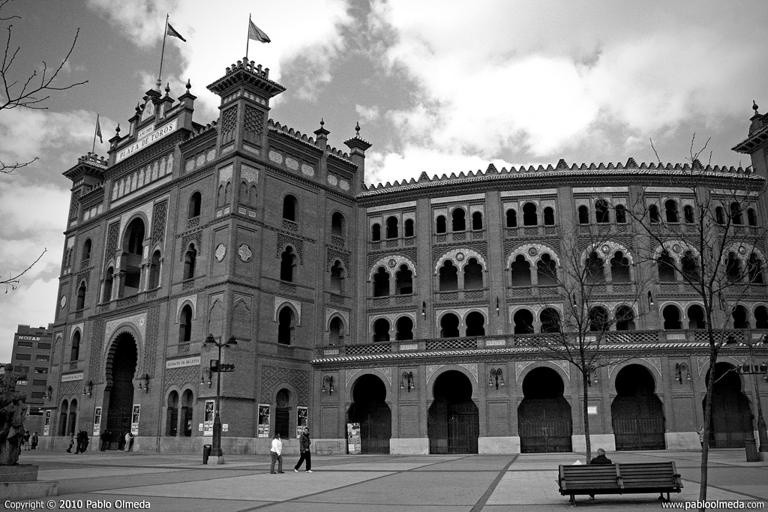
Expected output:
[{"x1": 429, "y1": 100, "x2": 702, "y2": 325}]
[
  {"x1": 745, "y1": 438, "x2": 760, "y2": 462},
  {"x1": 203, "y1": 444, "x2": 211, "y2": 464}
]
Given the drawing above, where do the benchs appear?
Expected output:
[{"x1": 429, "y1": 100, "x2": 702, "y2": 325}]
[{"x1": 556, "y1": 461, "x2": 682, "y2": 502}]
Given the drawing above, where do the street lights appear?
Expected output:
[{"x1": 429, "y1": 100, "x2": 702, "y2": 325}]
[
  {"x1": 738, "y1": 325, "x2": 767, "y2": 461},
  {"x1": 206, "y1": 333, "x2": 237, "y2": 465}
]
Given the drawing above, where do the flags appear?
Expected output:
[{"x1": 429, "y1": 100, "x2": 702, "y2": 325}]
[
  {"x1": 248, "y1": 13, "x2": 271, "y2": 43},
  {"x1": 96, "y1": 114, "x2": 103, "y2": 143},
  {"x1": 167, "y1": 23, "x2": 187, "y2": 42}
]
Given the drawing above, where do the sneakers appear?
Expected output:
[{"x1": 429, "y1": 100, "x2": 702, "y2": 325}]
[
  {"x1": 294, "y1": 468, "x2": 299, "y2": 473},
  {"x1": 271, "y1": 471, "x2": 284, "y2": 474},
  {"x1": 306, "y1": 470, "x2": 312, "y2": 472}
]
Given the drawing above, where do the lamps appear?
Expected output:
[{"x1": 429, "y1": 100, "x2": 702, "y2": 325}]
[
  {"x1": 138, "y1": 373, "x2": 147, "y2": 392},
  {"x1": 200, "y1": 368, "x2": 212, "y2": 388},
  {"x1": 488, "y1": 368, "x2": 505, "y2": 389},
  {"x1": 674, "y1": 362, "x2": 690, "y2": 383},
  {"x1": 321, "y1": 375, "x2": 335, "y2": 395},
  {"x1": 42, "y1": 386, "x2": 53, "y2": 402},
  {"x1": 83, "y1": 380, "x2": 93, "y2": 398},
  {"x1": 584, "y1": 364, "x2": 599, "y2": 385},
  {"x1": 400, "y1": 372, "x2": 414, "y2": 392}
]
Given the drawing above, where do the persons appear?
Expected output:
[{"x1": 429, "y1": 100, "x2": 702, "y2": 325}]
[
  {"x1": 101, "y1": 430, "x2": 134, "y2": 451},
  {"x1": 696, "y1": 425, "x2": 704, "y2": 448},
  {"x1": 66, "y1": 430, "x2": 89, "y2": 454},
  {"x1": 21, "y1": 431, "x2": 38, "y2": 451},
  {"x1": 590, "y1": 448, "x2": 612, "y2": 501},
  {"x1": 0, "y1": 363, "x2": 28, "y2": 463},
  {"x1": 294, "y1": 427, "x2": 313, "y2": 473},
  {"x1": 270, "y1": 433, "x2": 285, "y2": 474}
]
